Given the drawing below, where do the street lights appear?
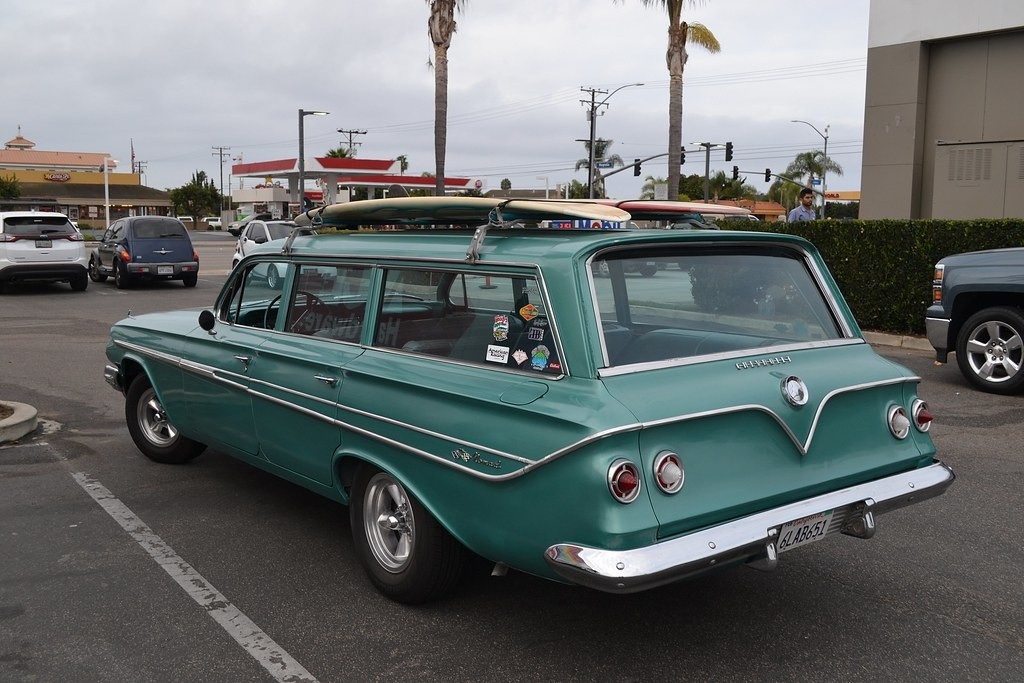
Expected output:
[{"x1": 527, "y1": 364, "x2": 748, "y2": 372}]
[
  {"x1": 589, "y1": 83, "x2": 645, "y2": 198},
  {"x1": 790, "y1": 120, "x2": 828, "y2": 219},
  {"x1": 298, "y1": 109, "x2": 330, "y2": 215},
  {"x1": 105, "y1": 157, "x2": 119, "y2": 229}
]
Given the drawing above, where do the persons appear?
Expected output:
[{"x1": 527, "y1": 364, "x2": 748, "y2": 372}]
[{"x1": 787, "y1": 187, "x2": 817, "y2": 223}]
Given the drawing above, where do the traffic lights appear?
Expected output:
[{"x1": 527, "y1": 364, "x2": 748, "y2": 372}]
[
  {"x1": 733, "y1": 166, "x2": 738, "y2": 179},
  {"x1": 634, "y1": 159, "x2": 641, "y2": 176},
  {"x1": 725, "y1": 142, "x2": 733, "y2": 161},
  {"x1": 765, "y1": 169, "x2": 771, "y2": 182},
  {"x1": 681, "y1": 146, "x2": 685, "y2": 164}
]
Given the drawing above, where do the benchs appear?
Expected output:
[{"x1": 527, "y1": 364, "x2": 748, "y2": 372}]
[
  {"x1": 313, "y1": 314, "x2": 526, "y2": 351},
  {"x1": 616, "y1": 328, "x2": 797, "y2": 368},
  {"x1": 403, "y1": 324, "x2": 631, "y2": 367}
]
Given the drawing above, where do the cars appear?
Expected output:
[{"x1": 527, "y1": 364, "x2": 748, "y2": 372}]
[
  {"x1": 925, "y1": 248, "x2": 1024, "y2": 394},
  {"x1": 0, "y1": 209, "x2": 90, "y2": 292},
  {"x1": 87, "y1": 215, "x2": 199, "y2": 289},
  {"x1": 666, "y1": 212, "x2": 759, "y2": 272},
  {"x1": 231, "y1": 219, "x2": 337, "y2": 290},
  {"x1": 541, "y1": 216, "x2": 659, "y2": 277},
  {"x1": 201, "y1": 216, "x2": 221, "y2": 231},
  {"x1": 105, "y1": 195, "x2": 954, "y2": 601},
  {"x1": 227, "y1": 213, "x2": 284, "y2": 236},
  {"x1": 177, "y1": 217, "x2": 193, "y2": 222}
]
